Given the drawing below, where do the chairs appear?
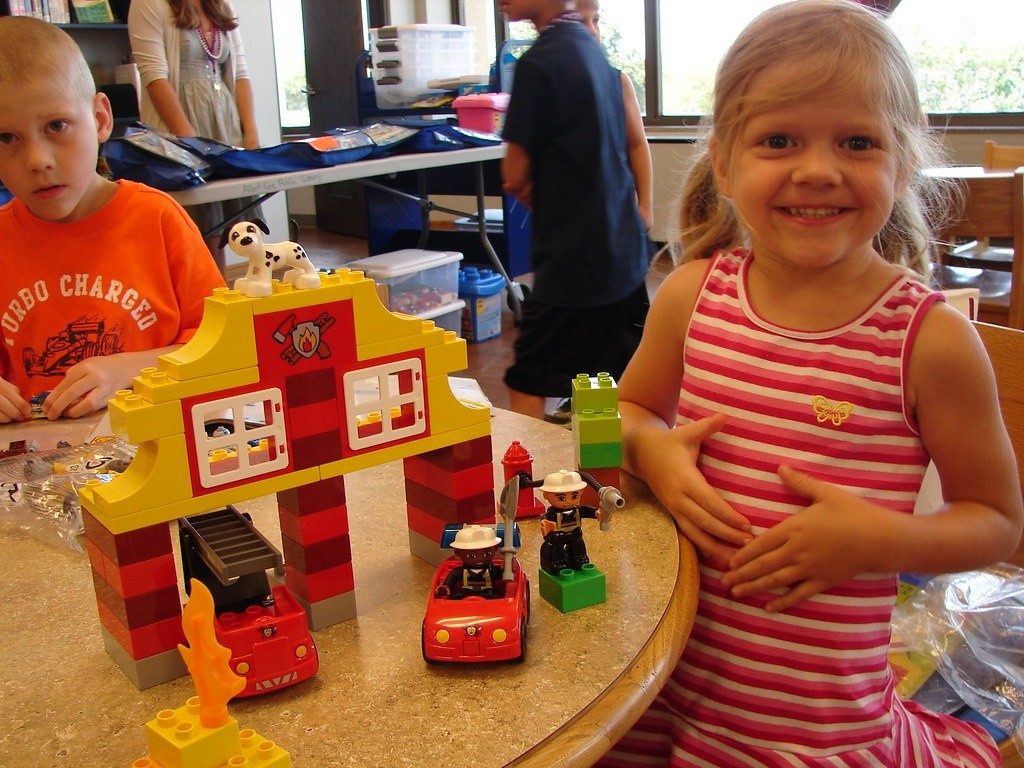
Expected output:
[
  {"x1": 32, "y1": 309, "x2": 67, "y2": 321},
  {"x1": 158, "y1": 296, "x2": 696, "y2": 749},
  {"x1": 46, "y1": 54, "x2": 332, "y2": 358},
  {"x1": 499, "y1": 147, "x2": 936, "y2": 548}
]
[
  {"x1": 887, "y1": 163, "x2": 1024, "y2": 330},
  {"x1": 931, "y1": 140, "x2": 1024, "y2": 326}
]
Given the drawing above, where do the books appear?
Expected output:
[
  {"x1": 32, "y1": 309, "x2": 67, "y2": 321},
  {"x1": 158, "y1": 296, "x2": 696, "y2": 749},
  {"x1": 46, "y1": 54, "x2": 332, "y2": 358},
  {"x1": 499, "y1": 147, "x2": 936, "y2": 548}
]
[{"x1": 1, "y1": 0, "x2": 115, "y2": 26}]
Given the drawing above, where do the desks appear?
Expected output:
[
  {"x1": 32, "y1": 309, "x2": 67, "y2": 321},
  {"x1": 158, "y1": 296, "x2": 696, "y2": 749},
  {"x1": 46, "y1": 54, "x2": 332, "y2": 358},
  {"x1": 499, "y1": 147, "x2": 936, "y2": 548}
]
[
  {"x1": 0, "y1": 407, "x2": 700, "y2": 768},
  {"x1": 169, "y1": 144, "x2": 525, "y2": 331}
]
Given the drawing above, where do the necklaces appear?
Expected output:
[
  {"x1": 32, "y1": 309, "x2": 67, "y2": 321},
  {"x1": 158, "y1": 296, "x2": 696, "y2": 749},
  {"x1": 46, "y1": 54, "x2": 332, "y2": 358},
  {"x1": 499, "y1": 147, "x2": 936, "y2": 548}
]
[{"x1": 197, "y1": 27, "x2": 222, "y2": 59}]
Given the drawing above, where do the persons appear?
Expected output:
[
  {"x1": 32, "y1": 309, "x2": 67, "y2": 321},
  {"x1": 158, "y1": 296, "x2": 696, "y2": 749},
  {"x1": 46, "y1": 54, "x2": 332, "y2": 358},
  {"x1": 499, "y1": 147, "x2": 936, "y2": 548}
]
[
  {"x1": 614, "y1": 0, "x2": 1024, "y2": 768},
  {"x1": 127, "y1": 0, "x2": 267, "y2": 267},
  {"x1": 1, "y1": 16, "x2": 231, "y2": 425},
  {"x1": 498, "y1": 0, "x2": 655, "y2": 429},
  {"x1": 539, "y1": 469, "x2": 612, "y2": 574},
  {"x1": 441, "y1": 524, "x2": 508, "y2": 600}
]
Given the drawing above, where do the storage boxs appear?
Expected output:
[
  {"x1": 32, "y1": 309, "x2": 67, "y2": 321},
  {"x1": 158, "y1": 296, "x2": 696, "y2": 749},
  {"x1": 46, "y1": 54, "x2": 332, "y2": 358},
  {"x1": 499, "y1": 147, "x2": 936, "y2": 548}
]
[
  {"x1": 416, "y1": 301, "x2": 468, "y2": 337},
  {"x1": 454, "y1": 209, "x2": 503, "y2": 234},
  {"x1": 369, "y1": 24, "x2": 513, "y2": 134},
  {"x1": 344, "y1": 246, "x2": 463, "y2": 319},
  {"x1": 461, "y1": 273, "x2": 506, "y2": 342}
]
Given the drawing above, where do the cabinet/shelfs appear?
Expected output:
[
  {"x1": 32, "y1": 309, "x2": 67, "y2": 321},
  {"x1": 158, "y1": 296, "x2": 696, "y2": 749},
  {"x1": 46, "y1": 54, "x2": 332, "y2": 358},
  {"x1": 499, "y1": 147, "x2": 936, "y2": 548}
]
[{"x1": 52, "y1": 23, "x2": 140, "y2": 126}]
